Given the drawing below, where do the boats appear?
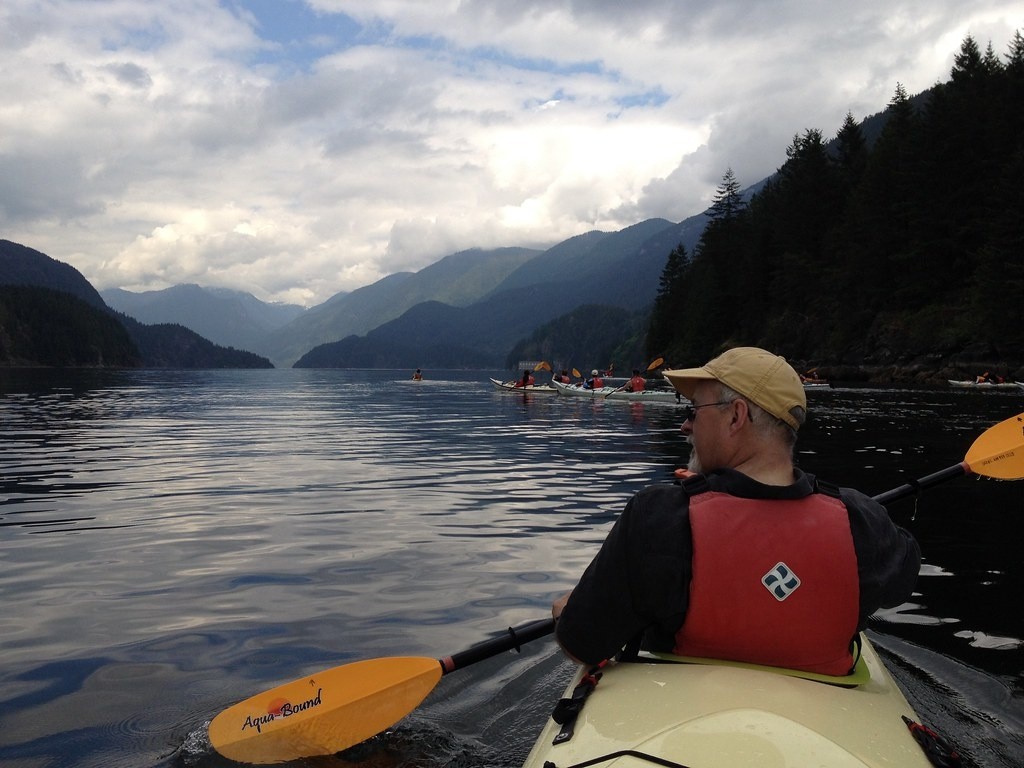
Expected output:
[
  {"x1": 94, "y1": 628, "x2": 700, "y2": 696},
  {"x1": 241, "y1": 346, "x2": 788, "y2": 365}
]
[
  {"x1": 394, "y1": 380, "x2": 446, "y2": 386},
  {"x1": 1015, "y1": 381, "x2": 1024, "y2": 392},
  {"x1": 948, "y1": 380, "x2": 1018, "y2": 393},
  {"x1": 490, "y1": 378, "x2": 557, "y2": 394},
  {"x1": 552, "y1": 379, "x2": 688, "y2": 403},
  {"x1": 802, "y1": 383, "x2": 832, "y2": 391}
]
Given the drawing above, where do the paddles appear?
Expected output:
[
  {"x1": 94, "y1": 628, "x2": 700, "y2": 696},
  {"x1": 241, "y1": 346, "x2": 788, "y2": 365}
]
[
  {"x1": 598, "y1": 357, "x2": 663, "y2": 399},
  {"x1": 508, "y1": 362, "x2": 544, "y2": 391},
  {"x1": 572, "y1": 368, "x2": 588, "y2": 382},
  {"x1": 208, "y1": 408, "x2": 1024, "y2": 765},
  {"x1": 544, "y1": 362, "x2": 559, "y2": 378}
]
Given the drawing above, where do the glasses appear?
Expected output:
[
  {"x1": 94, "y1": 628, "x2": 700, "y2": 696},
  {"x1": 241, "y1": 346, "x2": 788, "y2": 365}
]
[{"x1": 685, "y1": 402, "x2": 733, "y2": 422}]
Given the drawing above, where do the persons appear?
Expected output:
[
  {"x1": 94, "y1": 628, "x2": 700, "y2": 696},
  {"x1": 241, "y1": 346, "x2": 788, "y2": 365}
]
[
  {"x1": 552, "y1": 370, "x2": 570, "y2": 384},
  {"x1": 519, "y1": 370, "x2": 535, "y2": 386},
  {"x1": 799, "y1": 372, "x2": 818, "y2": 383},
  {"x1": 622, "y1": 369, "x2": 646, "y2": 392},
  {"x1": 551, "y1": 347, "x2": 923, "y2": 674},
  {"x1": 584, "y1": 369, "x2": 604, "y2": 389},
  {"x1": 976, "y1": 371, "x2": 1005, "y2": 383},
  {"x1": 413, "y1": 369, "x2": 423, "y2": 380}
]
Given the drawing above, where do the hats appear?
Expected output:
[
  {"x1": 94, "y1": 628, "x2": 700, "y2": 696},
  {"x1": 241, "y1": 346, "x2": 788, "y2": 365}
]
[
  {"x1": 592, "y1": 370, "x2": 598, "y2": 375},
  {"x1": 661, "y1": 347, "x2": 806, "y2": 433}
]
